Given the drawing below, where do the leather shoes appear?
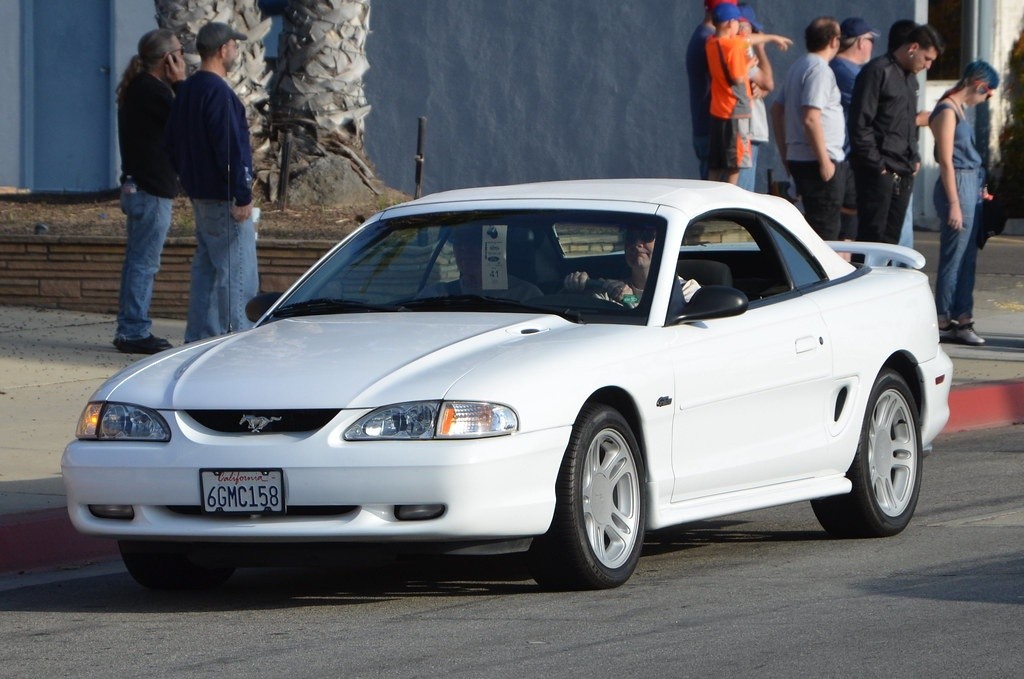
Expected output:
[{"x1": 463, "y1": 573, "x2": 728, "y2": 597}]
[{"x1": 112, "y1": 335, "x2": 172, "y2": 354}]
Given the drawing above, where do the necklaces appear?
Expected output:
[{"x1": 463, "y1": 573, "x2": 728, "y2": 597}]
[{"x1": 947, "y1": 95, "x2": 965, "y2": 119}]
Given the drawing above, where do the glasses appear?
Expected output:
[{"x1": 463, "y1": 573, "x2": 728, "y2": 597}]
[
  {"x1": 164, "y1": 44, "x2": 186, "y2": 60},
  {"x1": 974, "y1": 81, "x2": 992, "y2": 101}
]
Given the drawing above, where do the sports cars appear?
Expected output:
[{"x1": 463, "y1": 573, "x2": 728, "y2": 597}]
[{"x1": 60, "y1": 178, "x2": 954, "y2": 586}]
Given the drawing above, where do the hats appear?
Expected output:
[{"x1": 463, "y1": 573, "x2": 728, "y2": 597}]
[
  {"x1": 738, "y1": 4, "x2": 763, "y2": 29},
  {"x1": 712, "y1": 4, "x2": 740, "y2": 25},
  {"x1": 198, "y1": 22, "x2": 248, "y2": 48},
  {"x1": 705, "y1": 0, "x2": 738, "y2": 10},
  {"x1": 840, "y1": 18, "x2": 880, "y2": 43}
]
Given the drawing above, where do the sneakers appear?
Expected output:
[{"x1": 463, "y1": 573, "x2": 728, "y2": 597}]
[{"x1": 939, "y1": 323, "x2": 985, "y2": 347}]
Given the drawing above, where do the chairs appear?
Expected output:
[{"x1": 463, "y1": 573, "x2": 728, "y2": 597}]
[{"x1": 680, "y1": 260, "x2": 732, "y2": 287}]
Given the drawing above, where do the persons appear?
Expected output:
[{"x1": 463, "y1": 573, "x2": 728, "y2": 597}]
[
  {"x1": 115, "y1": 28, "x2": 186, "y2": 354},
  {"x1": 685, "y1": 0, "x2": 1000, "y2": 346},
  {"x1": 175, "y1": 23, "x2": 261, "y2": 344},
  {"x1": 413, "y1": 215, "x2": 704, "y2": 309}
]
